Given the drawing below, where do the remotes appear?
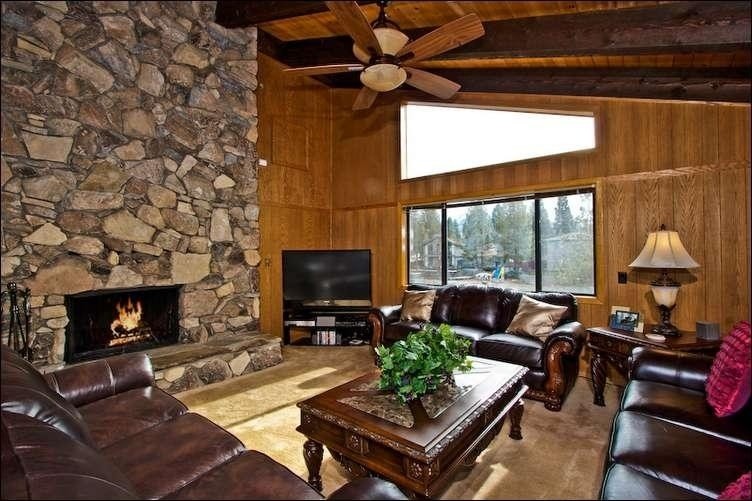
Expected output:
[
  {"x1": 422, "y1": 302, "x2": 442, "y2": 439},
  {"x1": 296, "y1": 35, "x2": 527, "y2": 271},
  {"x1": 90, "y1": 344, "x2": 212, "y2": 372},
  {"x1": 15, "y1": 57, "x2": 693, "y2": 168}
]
[{"x1": 645, "y1": 334, "x2": 666, "y2": 341}]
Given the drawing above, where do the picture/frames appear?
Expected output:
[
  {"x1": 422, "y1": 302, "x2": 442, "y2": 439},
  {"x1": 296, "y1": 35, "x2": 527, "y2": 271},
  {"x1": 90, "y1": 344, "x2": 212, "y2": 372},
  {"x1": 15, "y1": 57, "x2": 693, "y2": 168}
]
[{"x1": 614, "y1": 311, "x2": 640, "y2": 329}]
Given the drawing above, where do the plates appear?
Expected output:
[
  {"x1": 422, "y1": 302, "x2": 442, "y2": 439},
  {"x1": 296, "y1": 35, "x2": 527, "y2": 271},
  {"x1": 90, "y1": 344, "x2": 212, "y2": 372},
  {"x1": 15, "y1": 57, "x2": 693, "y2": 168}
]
[{"x1": 645, "y1": 333, "x2": 665, "y2": 342}]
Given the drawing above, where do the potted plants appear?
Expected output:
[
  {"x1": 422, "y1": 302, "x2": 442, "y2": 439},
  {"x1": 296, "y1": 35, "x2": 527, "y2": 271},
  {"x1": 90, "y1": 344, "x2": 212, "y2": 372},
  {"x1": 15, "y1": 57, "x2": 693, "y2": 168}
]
[{"x1": 371, "y1": 323, "x2": 472, "y2": 405}]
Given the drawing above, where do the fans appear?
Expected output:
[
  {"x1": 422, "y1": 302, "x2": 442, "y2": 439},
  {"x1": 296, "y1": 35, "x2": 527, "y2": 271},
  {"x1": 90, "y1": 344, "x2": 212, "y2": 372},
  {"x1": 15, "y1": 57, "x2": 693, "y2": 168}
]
[{"x1": 279, "y1": 1, "x2": 485, "y2": 112}]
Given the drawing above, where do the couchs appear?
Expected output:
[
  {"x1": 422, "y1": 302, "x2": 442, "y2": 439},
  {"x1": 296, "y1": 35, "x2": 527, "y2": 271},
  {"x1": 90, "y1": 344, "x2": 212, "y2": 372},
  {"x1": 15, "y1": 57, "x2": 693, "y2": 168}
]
[
  {"x1": 599, "y1": 348, "x2": 752, "y2": 501},
  {"x1": 0, "y1": 344, "x2": 410, "y2": 501},
  {"x1": 369, "y1": 284, "x2": 587, "y2": 412}
]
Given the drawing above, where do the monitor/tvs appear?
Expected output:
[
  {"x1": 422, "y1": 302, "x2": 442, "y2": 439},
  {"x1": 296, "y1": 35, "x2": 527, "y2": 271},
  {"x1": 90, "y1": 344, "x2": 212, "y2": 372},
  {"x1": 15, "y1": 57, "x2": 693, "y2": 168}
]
[{"x1": 282, "y1": 249, "x2": 371, "y2": 306}]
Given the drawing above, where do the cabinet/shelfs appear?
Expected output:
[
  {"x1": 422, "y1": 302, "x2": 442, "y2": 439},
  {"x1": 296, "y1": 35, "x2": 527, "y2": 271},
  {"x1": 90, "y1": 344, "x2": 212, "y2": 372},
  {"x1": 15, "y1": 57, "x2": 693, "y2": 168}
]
[{"x1": 282, "y1": 308, "x2": 376, "y2": 347}]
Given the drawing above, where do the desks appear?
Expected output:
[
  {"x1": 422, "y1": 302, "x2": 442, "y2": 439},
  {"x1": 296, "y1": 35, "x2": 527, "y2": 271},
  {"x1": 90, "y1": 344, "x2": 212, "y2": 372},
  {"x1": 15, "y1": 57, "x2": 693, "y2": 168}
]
[{"x1": 586, "y1": 324, "x2": 723, "y2": 408}]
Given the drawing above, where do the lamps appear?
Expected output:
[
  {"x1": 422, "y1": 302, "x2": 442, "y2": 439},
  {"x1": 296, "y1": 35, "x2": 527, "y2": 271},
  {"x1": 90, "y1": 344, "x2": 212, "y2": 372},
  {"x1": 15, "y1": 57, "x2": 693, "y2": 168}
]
[{"x1": 627, "y1": 222, "x2": 701, "y2": 339}]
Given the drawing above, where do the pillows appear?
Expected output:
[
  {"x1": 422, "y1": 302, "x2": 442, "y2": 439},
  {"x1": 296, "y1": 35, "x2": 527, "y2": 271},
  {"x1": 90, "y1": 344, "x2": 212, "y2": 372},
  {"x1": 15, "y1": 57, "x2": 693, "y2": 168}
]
[
  {"x1": 716, "y1": 470, "x2": 751, "y2": 501},
  {"x1": 505, "y1": 295, "x2": 568, "y2": 344},
  {"x1": 399, "y1": 289, "x2": 436, "y2": 322},
  {"x1": 705, "y1": 321, "x2": 752, "y2": 419}
]
